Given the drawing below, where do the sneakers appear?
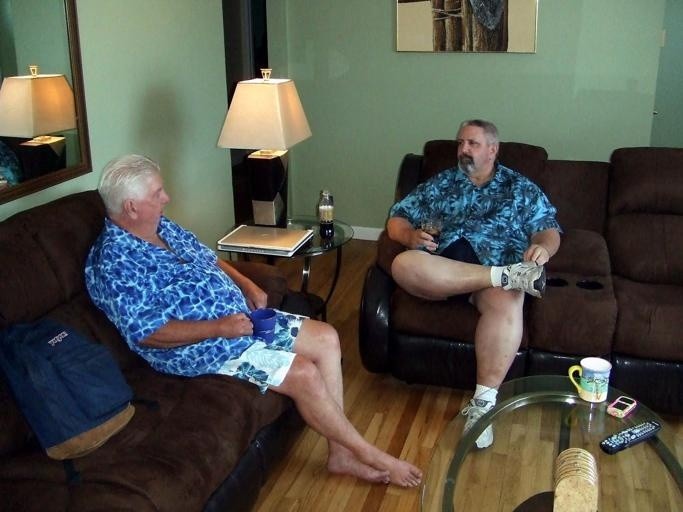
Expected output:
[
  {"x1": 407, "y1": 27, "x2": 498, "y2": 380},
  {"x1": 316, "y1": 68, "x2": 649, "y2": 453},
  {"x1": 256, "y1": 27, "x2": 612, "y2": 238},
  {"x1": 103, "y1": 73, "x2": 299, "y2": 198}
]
[
  {"x1": 501, "y1": 261, "x2": 547, "y2": 299},
  {"x1": 460, "y1": 399, "x2": 494, "y2": 450}
]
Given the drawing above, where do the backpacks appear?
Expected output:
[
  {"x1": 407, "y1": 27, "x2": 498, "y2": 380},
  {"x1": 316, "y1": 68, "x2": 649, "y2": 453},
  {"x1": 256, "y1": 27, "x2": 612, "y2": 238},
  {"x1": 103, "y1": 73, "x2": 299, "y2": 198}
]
[{"x1": 0, "y1": 315, "x2": 159, "y2": 483}]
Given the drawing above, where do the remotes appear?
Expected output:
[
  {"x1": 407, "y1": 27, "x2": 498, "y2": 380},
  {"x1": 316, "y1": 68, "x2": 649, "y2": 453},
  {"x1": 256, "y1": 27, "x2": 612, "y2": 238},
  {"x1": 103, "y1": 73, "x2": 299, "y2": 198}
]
[{"x1": 600, "y1": 420, "x2": 661, "y2": 453}]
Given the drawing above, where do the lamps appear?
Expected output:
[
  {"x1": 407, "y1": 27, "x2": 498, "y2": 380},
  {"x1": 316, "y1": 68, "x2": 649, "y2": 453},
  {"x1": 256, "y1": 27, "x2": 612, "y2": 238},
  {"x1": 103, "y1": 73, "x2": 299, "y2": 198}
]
[
  {"x1": 0, "y1": 66, "x2": 78, "y2": 184},
  {"x1": 216, "y1": 68, "x2": 311, "y2": 226}
]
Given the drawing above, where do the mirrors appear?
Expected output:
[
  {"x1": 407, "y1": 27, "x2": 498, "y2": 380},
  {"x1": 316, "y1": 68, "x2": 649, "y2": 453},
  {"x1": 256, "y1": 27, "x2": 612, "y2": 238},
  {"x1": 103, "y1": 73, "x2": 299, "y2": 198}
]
[{"x1": 0, "y1": 1, "x2": 93, "y2": 209}]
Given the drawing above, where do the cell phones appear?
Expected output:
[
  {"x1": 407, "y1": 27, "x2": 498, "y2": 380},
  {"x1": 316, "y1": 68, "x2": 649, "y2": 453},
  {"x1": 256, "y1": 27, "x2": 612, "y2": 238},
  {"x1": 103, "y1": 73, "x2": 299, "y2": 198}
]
[{"x1": 606, "y1": 396, "x2": 637, "y2": 419}]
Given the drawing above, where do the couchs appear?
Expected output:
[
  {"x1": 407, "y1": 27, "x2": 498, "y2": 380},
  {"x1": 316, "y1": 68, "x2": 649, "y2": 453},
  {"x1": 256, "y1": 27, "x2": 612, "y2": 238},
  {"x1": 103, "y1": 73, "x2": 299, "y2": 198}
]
[
  {"x1": 357, "y1": 140, "x2": 682, "y2": 415},
  {"x1": 0, "y1": 192, "x2": 325, "y2": 510}
]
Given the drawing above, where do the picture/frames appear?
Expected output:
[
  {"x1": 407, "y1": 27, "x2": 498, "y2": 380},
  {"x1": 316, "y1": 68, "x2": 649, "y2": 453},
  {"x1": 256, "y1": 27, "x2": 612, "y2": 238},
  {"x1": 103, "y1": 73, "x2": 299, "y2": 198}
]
[{"x1": 396, "y1": 0, "x2": 538, "y2": 54}]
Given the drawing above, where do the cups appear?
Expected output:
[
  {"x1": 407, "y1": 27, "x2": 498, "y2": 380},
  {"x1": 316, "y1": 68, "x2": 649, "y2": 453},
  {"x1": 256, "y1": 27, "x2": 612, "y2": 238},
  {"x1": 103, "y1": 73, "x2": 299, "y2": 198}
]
[
  {"x1": 568, "y1": 357, "x2": 612, "y2": 403},
  {"x1": 249, "y1": 308, "x2": 276, "y2": 343},
  {"x1": 419, "y1": 213, "x2": 445, "y2": 254}
]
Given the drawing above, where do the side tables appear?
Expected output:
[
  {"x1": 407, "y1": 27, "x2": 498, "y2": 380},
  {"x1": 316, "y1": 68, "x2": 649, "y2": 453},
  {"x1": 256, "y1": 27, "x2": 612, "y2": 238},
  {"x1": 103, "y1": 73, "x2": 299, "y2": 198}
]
[{"x1": 222, "y1": 215, "x2": 354, "y2": 329}]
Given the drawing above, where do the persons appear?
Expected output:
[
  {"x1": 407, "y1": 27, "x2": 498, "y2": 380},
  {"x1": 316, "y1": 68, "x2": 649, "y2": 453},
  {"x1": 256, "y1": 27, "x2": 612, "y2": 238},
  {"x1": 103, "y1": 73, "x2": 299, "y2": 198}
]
[
  {"x1": 384, "y1": 118, "x2": 561, "y2": 449},
  {"x1": 84, "y1": 152, "x2": 423, "y2": 489}
]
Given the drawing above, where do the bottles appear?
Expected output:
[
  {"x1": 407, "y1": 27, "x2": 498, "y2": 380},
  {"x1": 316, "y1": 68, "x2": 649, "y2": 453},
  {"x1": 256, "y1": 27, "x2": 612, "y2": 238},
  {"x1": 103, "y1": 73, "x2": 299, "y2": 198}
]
[{"x1": 318, "y1": 189, "x2": 335, "y2": 241}]
